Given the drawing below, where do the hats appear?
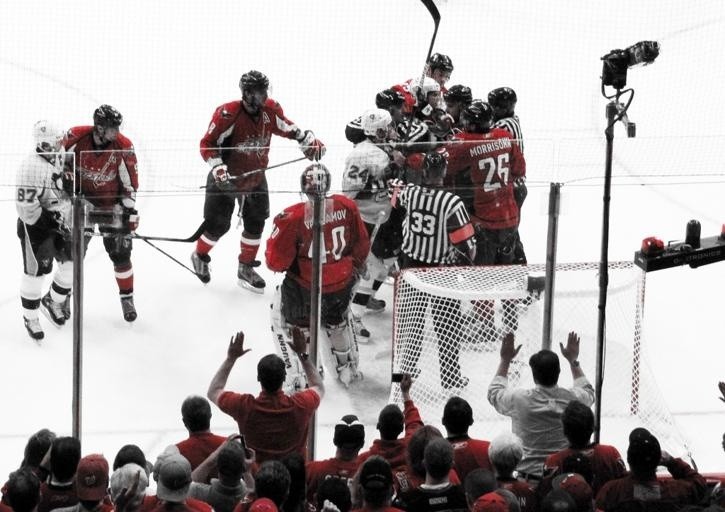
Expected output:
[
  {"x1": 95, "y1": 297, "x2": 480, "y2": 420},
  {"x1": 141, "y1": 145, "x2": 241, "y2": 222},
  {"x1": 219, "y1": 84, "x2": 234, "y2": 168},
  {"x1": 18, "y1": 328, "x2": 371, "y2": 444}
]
[
  {"x1": 529, "y1": 350, "x2": 560, "y2": 374},
  {"x1": 551, "y1": 473, "x2": 591, "y2": 498},
  {"x1": 156, "y1": 455, "x2": 193, "y2": 502},
  {"x1": 626, "y1": 427, "x2": 662, "y2": 468},
  {"x1": 335, "y1": 412, "x2": 366, "y2": 442},
  {"x1": 76, "y1": 454, "x2": 109, "y2": 501},
  {"x1": 473, "y1": 492, "x2": 507, "y2": 512}
]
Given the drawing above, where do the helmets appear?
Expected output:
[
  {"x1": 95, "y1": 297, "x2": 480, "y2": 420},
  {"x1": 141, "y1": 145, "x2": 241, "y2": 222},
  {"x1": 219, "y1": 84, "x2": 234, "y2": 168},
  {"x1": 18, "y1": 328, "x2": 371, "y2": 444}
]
[
  {"x1": 375, "y1": 89, "x2": 406, "y2": 108},
  {"x1": 93, "y1": 103, "x2": 122, "y2": 126},
  {"x1": 443, "y1": 83, "x2": 472, "y2": 103},
  {"x1": 238, "y1": 70, "x2": 270, "y2": 92},
  {"x1": 428, "y1": 52, "x2": 455, "y2": 72},
  {"x1": 31, "y1": 116, "x2": 66, "y2": 150},
  {"x1": 299, "y1": 164, "x2": 331, "y2": 193},
  {"x1": 408, "y1": 77, "x2": 441, "y2": 100},
  {"x1": 423, "y1": 152, "x2": 447, "y2": 173},
  {"x1": 361, "y1": 108, "x2": 392, "y2": 135},
  {"x1": 487, "y1": 86, "x2": 518, "y2": 107},
  {"x1": 461, "y1": 98, "x2": 493, "y2": 127}
]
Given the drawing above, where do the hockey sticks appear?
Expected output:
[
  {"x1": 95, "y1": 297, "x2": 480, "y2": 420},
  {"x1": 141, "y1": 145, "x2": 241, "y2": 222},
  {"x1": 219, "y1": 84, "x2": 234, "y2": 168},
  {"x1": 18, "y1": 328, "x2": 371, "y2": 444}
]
[
  {"x1": 83, "y1": 221, "x2": 210, "y2": 242},
  {"x1": 132, "y1": 228, "x2": 211, "y2": 282},
  {"x1": 401, "y1": 1, "x2": 441, "y2": 156},
  {"x1": 198, "y1": 156, "x2": 309, "y2": 188}
]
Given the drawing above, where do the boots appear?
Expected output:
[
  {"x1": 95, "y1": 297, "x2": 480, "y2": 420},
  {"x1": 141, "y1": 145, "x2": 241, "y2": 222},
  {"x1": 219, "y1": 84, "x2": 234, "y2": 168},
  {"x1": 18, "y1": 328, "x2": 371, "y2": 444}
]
[
  {"x1": 523, "y1": 276, "x2": 546, "y2": 304},
  {"x1": 235, "y1": 262, "x2": 268, "y2": 288},
  {"x1": 120, "y1": 296, "x2": 138, "y2": 322},
  {"x1": 351, "y1": 318, "x2": 371, "y2": 337},
  {"x1": 443, "y1": 374, "x2": 470, "y2": 388},
  {"x1": 367, "y1": 295, "x2": 386, "y2": 310},
  {"x1": 191, "y1": 251, "x2": 212, "y2": 283},
  {"x1": 42, "y1": 290, "x2": 71, "y2": 324},
  {"x1": 461, "y1": 305, "x2": 516, "y2": 342},
  {"x1": 23, "y1": 313, "x2": 45, "y2": 340}
]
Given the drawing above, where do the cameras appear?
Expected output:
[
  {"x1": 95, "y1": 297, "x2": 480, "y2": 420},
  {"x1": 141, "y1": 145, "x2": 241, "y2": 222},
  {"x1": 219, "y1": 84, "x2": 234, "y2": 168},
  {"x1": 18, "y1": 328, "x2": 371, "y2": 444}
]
[
  {"x1": 229, "y1": 435, "x2": 250, "y2": 459},
  {"x1": 391, "y1": 373, "x2": 408, "y2": 383}
]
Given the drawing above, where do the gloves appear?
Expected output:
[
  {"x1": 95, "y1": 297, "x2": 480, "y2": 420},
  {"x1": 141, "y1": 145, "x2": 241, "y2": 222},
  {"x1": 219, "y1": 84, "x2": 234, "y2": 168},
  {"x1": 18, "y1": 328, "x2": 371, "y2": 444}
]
[
  {"x1": 298, "y1": 129, "x2": 326, "y2": 161},
  {"x1": 211, "y1": 164, "x2": 230, "y2": 190},
  {"x1": 122, "y1": 206, "x2": 140, "y2": 231},
  {"x1": 54, "y1": 215, "x2": 71, "y2": 241}
]
